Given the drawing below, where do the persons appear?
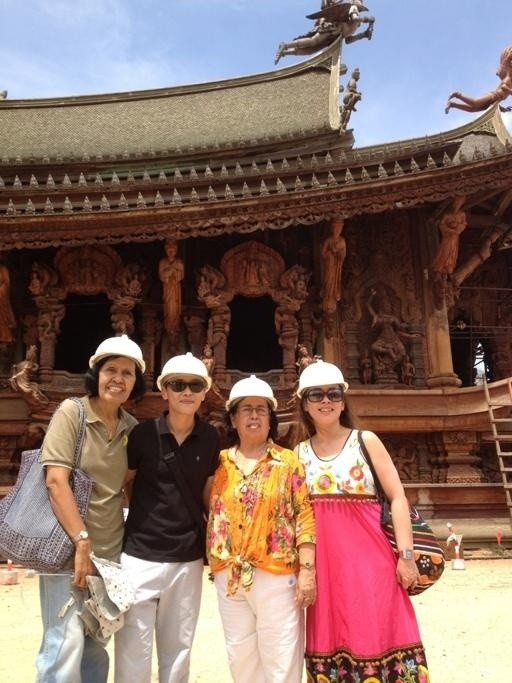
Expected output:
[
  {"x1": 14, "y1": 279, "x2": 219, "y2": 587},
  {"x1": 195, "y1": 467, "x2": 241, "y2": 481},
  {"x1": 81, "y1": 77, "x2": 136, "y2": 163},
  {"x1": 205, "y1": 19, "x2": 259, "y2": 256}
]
[
  {"x1": 275, "y1": 0, "x2": 375, "y2": 65},
  {"x1": 319, "y1": 220, "x2": 346, "y2": 307},
  {"x1": 0, "y1": 266, "x2": 18, "y2": 344},
  {"x1": 293, "y1": 362, "x2": 430, "y2": 683},
  {"x1": 206, "y1": 373, "x2": 317, "y2": 683},
  {"x1": 36, "y1": 334, "x2": 147, "y2": 683},
  {"x1": 114, "y1": 352, "x2": 221, "y2": 683},
  {"x1": 366, "y1": 291, "x2": 406, "y2": 359},
  {"x1": 343, "y1": 67, "x2": 361, "y2": 112},
  {"x1": 431, "y1": 195, "x2": 468, "y2": 275},
  {"x1": 157, "y1": 239, "x2": 185, "y2": 337},
  {"x1": 445, "y1": 45, "x2": 512, "y2": 112}
]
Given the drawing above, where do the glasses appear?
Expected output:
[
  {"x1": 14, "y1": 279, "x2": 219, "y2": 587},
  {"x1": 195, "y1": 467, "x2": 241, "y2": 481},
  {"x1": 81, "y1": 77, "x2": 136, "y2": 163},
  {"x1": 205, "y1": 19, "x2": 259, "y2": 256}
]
[
  {"x1": 302, "y1": 388, "x2": 344, "y2": 403},
  {"x1": 164, "y1": 379, "x2": 206, "y2": 394}
]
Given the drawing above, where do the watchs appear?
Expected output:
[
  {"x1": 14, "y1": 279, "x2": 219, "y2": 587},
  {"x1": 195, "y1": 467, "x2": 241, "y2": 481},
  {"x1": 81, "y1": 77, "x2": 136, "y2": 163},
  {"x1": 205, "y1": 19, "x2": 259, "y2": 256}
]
[
  {"x1": 299, "y1": 562, "x2": 315, "y2": 570},
  {"x1": 398, "y1": 549, "x2": 415, "y2": 560},
  {"x1": 70, "y1": 530, "x2": 90, "y2": 544}
]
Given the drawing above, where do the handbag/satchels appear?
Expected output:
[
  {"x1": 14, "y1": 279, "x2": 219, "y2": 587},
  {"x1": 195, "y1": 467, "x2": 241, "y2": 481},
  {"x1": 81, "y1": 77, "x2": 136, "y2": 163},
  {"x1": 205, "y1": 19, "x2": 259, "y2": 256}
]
[
  {"x1": 382, "y1": 503, "x2": 445, "y2": 595},
  {"x1": 0, "y1": 449, "x2": 97, "y2": 573}
]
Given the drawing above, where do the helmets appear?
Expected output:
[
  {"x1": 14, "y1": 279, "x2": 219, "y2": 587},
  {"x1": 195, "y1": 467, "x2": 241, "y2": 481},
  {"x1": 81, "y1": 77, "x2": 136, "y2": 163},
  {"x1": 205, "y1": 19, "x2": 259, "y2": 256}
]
[
  {"x1": 89, "y1": 334, "x2": 146, "y2": 375},
  {"x1": 224, "y1": 375, "x2": 278, "y2": 412},
  {"x1": 156, "y1": 352, "x2": 213, "y2": 393},
  {"x1": 295, "y1": 358, "x2": 349, "y2": 400}
]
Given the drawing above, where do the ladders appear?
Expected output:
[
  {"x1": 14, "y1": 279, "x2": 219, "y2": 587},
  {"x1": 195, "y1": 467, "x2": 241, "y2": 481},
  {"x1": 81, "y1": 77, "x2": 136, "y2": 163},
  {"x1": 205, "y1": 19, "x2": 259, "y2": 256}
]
[{"x1": 481, "y1": 372, "x2": 512, "y2": 515}]
